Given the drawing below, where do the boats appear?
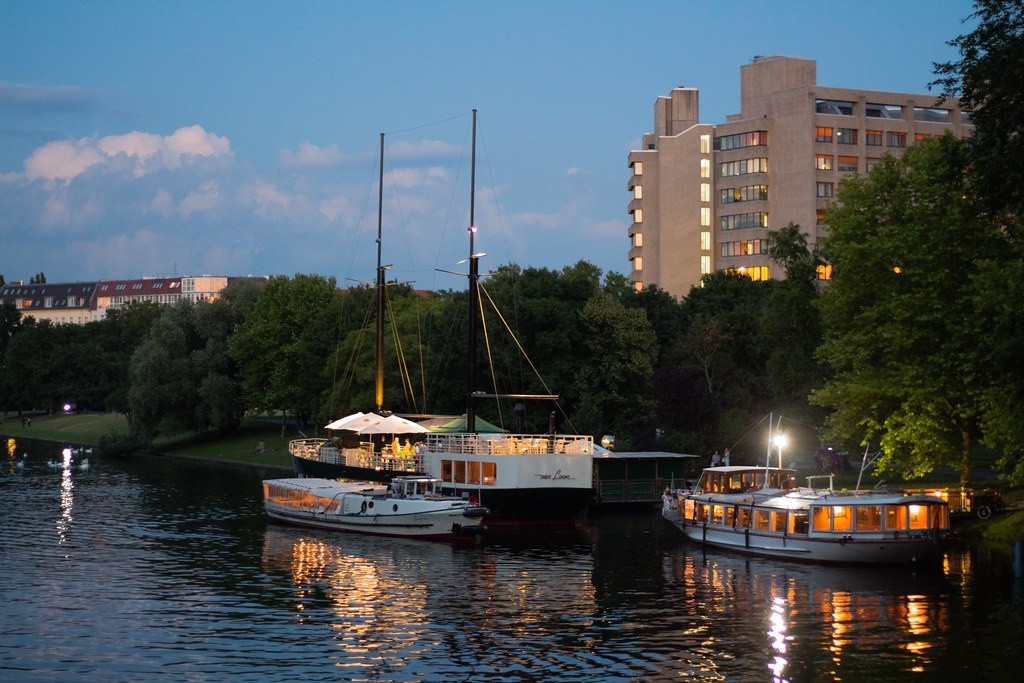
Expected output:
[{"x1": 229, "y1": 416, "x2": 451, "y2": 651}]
[
  {"x1": 261, "y1": 475, "x2": 493, "y2": 543},
  {"x1": 661, "y1": 411, "x2": 961, "y2": 568}
]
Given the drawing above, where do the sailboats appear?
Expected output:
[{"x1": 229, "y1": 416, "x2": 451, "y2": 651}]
[{"x1": 288, "y1": 111, "x2": 597, "y2": 520}]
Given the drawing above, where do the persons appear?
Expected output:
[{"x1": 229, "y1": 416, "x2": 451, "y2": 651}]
[
  {"x1": 22, "y1": 416, "x2": 30, "y2": 427},
  {"x1": 712, "y1": 447, "x2": 731, "y2": 466},
  {"x1": 375, "y1": 436, "x2": 425, "y2": 470}
]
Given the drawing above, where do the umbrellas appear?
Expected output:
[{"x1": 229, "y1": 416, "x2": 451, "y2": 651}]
[{"x1": 324, "y1": 412, "x2": 430, "y2": 451}]
